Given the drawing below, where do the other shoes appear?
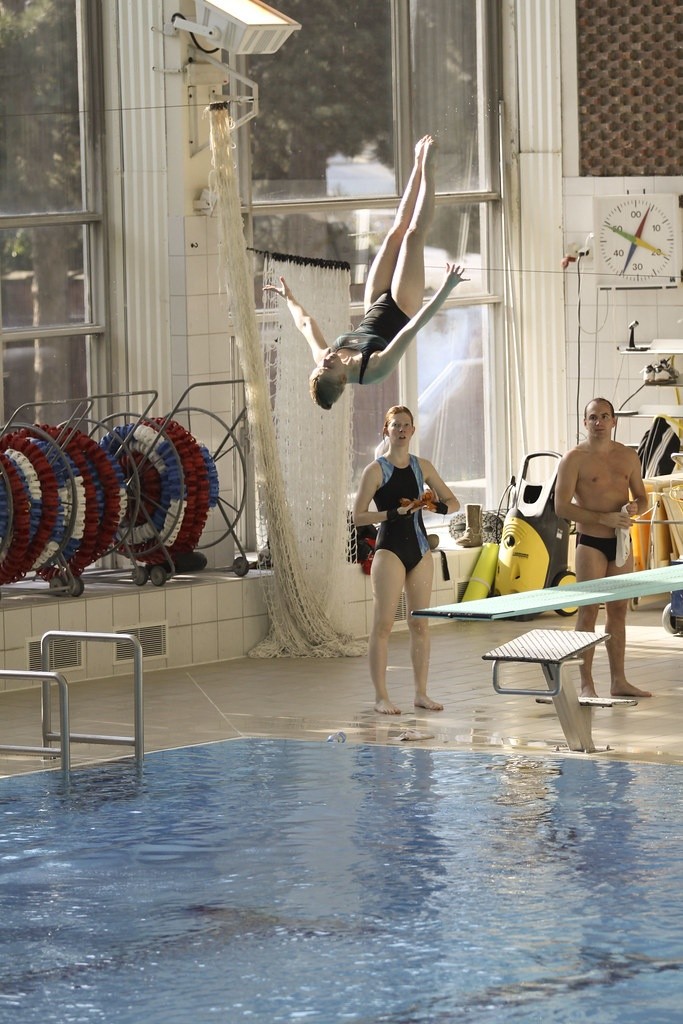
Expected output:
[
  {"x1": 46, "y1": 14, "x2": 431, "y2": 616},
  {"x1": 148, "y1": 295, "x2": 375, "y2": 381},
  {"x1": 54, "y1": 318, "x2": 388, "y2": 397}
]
[{"x1": 428, "y1": 534, "x2": 439, "y2": 550}]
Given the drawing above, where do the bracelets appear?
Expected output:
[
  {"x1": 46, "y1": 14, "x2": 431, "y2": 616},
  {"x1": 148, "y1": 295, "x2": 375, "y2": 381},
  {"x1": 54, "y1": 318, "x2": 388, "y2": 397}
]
[
  {"x1": 387, "y1": 509, "x2": 398, "y2": 520},
  {"x1": 435, "y1": 502, "x2": 448, "y2": 515}
]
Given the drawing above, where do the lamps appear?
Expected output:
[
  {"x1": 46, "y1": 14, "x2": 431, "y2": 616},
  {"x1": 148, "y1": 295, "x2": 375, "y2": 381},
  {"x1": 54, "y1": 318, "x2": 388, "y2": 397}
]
[{"x1": 171, "y1": 0, "x2": 302, "y2": 54}]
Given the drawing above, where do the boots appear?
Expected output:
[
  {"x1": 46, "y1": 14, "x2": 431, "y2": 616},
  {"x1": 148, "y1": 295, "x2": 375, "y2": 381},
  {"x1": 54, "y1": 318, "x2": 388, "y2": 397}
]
[{"x1": 457, "y1": 503, "x2": 484, "y2": 546}]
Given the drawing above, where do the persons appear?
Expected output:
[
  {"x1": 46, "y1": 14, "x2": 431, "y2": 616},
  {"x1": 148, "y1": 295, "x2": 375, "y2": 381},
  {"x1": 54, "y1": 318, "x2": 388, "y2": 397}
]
[
  {"x1": 261, "y1": 135, "x2": 471, "y2": 411},
  {"x1": 353, "y1": 406, "x2": 460, "y2": 715},
  {"x1": 555, "y1": 398, "x2": 653, "y2": 698}
]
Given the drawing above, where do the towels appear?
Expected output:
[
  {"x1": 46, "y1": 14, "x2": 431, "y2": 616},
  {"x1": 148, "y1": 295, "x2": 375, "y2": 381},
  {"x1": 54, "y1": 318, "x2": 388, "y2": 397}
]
[{"x1": 613, "y1": 502, "x2": 631, "y2": 567}]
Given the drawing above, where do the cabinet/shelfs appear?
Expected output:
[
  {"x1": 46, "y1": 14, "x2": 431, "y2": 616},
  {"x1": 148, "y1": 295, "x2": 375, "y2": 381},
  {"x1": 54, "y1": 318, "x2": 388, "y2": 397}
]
[{"x1": 613, "y1": 339, "x2": 683, "y2": 419}]
